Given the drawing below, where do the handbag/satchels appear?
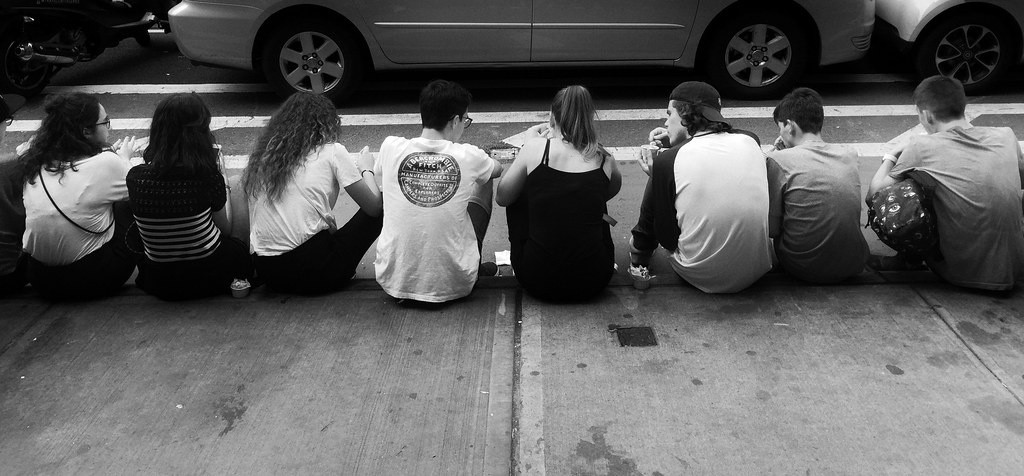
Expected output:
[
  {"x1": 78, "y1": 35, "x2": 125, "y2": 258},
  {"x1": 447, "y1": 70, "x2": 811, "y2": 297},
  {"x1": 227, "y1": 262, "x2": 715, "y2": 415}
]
[{"x1": 864, "y1": 171, "x2": 939, "y2": 254}]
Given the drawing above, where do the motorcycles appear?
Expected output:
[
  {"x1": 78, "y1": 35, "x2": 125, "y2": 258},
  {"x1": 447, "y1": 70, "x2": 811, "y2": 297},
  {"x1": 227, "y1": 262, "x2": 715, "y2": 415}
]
[{"x1": 5, "y1": 0, "x2": 157, "y2": 98}]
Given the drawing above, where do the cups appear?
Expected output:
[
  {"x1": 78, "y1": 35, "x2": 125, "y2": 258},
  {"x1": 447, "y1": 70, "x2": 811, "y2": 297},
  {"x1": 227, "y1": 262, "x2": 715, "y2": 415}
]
[
  {"x1": 641, "y1": 145, "x2": 659, "y2": 163},
  {"x1": 230, "y1": 282, "x2": 251, "y2": 298},
  {"x1": 634, "y1": 275, "x2": 651, "y2": 290}
]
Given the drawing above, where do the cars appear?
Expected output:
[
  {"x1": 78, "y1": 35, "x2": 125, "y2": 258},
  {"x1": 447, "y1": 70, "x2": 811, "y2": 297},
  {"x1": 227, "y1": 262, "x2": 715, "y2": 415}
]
[{"x1": 167, "y1": 0, "x2": 1024, "y2": 100}]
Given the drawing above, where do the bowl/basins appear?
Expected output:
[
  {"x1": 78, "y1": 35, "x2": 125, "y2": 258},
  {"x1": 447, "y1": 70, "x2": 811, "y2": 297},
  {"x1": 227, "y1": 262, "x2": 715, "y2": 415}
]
[{"x1": 761, "y1": 144, "x2": 775, "y2": 154}]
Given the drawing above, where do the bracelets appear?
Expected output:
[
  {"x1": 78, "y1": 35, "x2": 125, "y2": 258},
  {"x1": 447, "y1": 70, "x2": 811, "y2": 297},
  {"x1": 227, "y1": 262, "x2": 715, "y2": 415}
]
[{"x1": 361, "y1": 170, "x2": 374, "y2": 177}]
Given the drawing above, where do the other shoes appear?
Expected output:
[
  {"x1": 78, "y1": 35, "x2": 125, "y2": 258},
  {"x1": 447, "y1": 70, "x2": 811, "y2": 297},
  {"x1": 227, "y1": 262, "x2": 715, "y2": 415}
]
[
  {"x1": 628, "y1": 235, "x2": 649, "y2": 267},
  {"x1": 480, "y1": 261, "x2": 500, "y2": 276},
  {"x1": 881, "y1": 255, "x2": 930, "y2": 271}
]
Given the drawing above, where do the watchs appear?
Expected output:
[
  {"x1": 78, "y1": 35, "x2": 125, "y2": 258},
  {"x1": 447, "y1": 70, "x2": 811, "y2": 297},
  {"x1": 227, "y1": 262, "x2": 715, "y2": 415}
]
[
  {"x1": 225, "y1": 185, "x2": 231, "y2": 193},
  {"x1": 882, "y1": 153, "x2": 898, "y2": 164}
]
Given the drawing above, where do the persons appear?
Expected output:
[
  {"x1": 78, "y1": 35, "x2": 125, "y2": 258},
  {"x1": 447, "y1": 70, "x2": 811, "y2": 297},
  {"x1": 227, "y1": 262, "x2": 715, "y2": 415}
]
[
  {"x1": 495, "y1": 85, "x2": 622, "y2": 304},
  {"x1": 126, "y1": 90, "x2": 250, "y2": 302},
  {"x1": 628, "y1": 81, "x2": 773, "y2": 294},
  {"x1": 865, "y1": 76, "x2": 1024, "y2": 294},
  {"x1": 237, "y1": 91, "x2": 384, "y2": 298},
  {"x1": 766, "y1": 88, "x2": 871, "y2": 285},
  {"x1": 374, "y1": 79, "x2": 504, "y2": 311},
  {"x1": 0, "y1": 93, "x2": 141, "y2": 301}
]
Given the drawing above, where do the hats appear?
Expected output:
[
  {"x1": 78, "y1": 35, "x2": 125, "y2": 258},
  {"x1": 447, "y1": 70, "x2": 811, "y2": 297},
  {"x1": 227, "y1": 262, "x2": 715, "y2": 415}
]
[{"x1": 669, "y1": 81, "x2": 730, "y2": 129}]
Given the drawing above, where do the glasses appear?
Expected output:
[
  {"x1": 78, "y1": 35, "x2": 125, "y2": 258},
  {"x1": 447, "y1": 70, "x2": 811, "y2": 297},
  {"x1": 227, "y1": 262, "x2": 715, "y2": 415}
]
[
  {"x1": 87, "y1": 120, "x2": 110, "y2": 129},
  {"x1": 449, "y1": 113, "x2": 473, "y2": 128}
]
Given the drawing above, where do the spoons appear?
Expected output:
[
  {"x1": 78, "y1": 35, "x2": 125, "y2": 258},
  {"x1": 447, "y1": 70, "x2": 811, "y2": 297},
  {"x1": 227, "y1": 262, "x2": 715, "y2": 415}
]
[{"x1": 649, "y1": 140, "x2": 657, "y2": 145}]
